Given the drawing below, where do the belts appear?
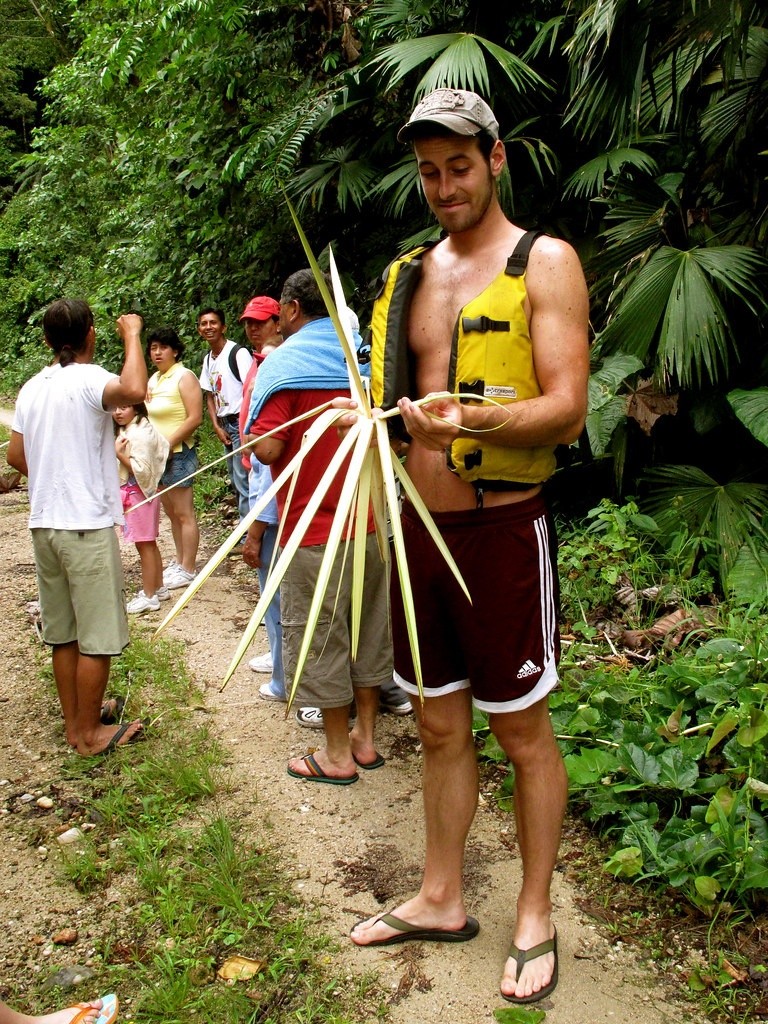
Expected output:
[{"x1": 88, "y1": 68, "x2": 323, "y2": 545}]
[{"x1": 218, "y1": 413, "x2": 240, "y2": 427}]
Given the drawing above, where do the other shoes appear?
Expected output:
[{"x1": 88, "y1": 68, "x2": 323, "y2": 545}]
[{"x1": 260, "y1": 618, "x2": 266, "y2": 625}]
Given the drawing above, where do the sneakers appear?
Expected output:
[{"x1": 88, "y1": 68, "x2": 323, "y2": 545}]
[
  {"x1": 378, "y1": 699, "x2": 413, "y2": 714},
  {"x1": 127, "y1": 594, "x2": 161, "y2": 614},
  {"x1": 295, "y1": 706, "x2": 357, "y2": 728},
  {"x1": 162, "y1": 562, "x2": 180, "y2": 578},
  {"x1": 248, "y1": 652, "x2": 274, "y2": 672},
  {"x1": 258, "y1": 684, "x2": 287, "y2": 702},
  {"x1": 138, "y1": 585, "x2": 171, "y2": 601},
  {"x1": 163, "y1": 568, "x2": 197, "y2": 589}
]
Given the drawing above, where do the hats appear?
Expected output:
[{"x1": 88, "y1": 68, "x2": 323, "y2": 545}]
[
  {"x1": 397, "y1": 87, "x2": 501, "y2": 147},
  {"x1": 239, "y1": 295, "x2": 279, "y2": 321}
]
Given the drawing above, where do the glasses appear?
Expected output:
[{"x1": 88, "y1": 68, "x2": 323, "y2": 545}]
[
  {"x1": 256, "y1": 360, "x2": 263, "y2": 368},
  {"x1": 274, "y1": 300, "x2": 293, "y2": 311}
]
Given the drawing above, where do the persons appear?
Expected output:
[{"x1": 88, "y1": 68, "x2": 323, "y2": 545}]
[
  {"x1": 243, "y1": 451, "x2": 289, "y2": 700},
  {"x1": 112, "y1": 296, "x2": 298, "y2": 613},
  {"x1": 5, "y1": 298, "x2": 148, "y2": 758},
  {"x1": 245, "y1": 268, "x2": 385, "y2": 786},
  {"x1": 329, "y1": 88, "x2": 589, "y2": 1003}
]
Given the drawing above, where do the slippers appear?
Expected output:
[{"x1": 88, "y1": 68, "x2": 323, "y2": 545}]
[
  {"x1": 351, "y1": 905, "x2": 480, "y2": 946},
  {"x1": 501, "y1": 924, "x2": 559, "y2": 1004},
  {"x1": 67, "y1": 994, "x2": 118, "y2": 1024},
  {"x1": 93, "y1": 716, "x2": 151, "y2": 756},
  {"x1": 100, "y1": 695, "x2": 125, "y2": 724},
  {"x1": 352, "y1": 749, "x2": 385, "y2": 770},
  {"x1": 287, "y1": 752, "x2": 359, "y2": 784}
]
